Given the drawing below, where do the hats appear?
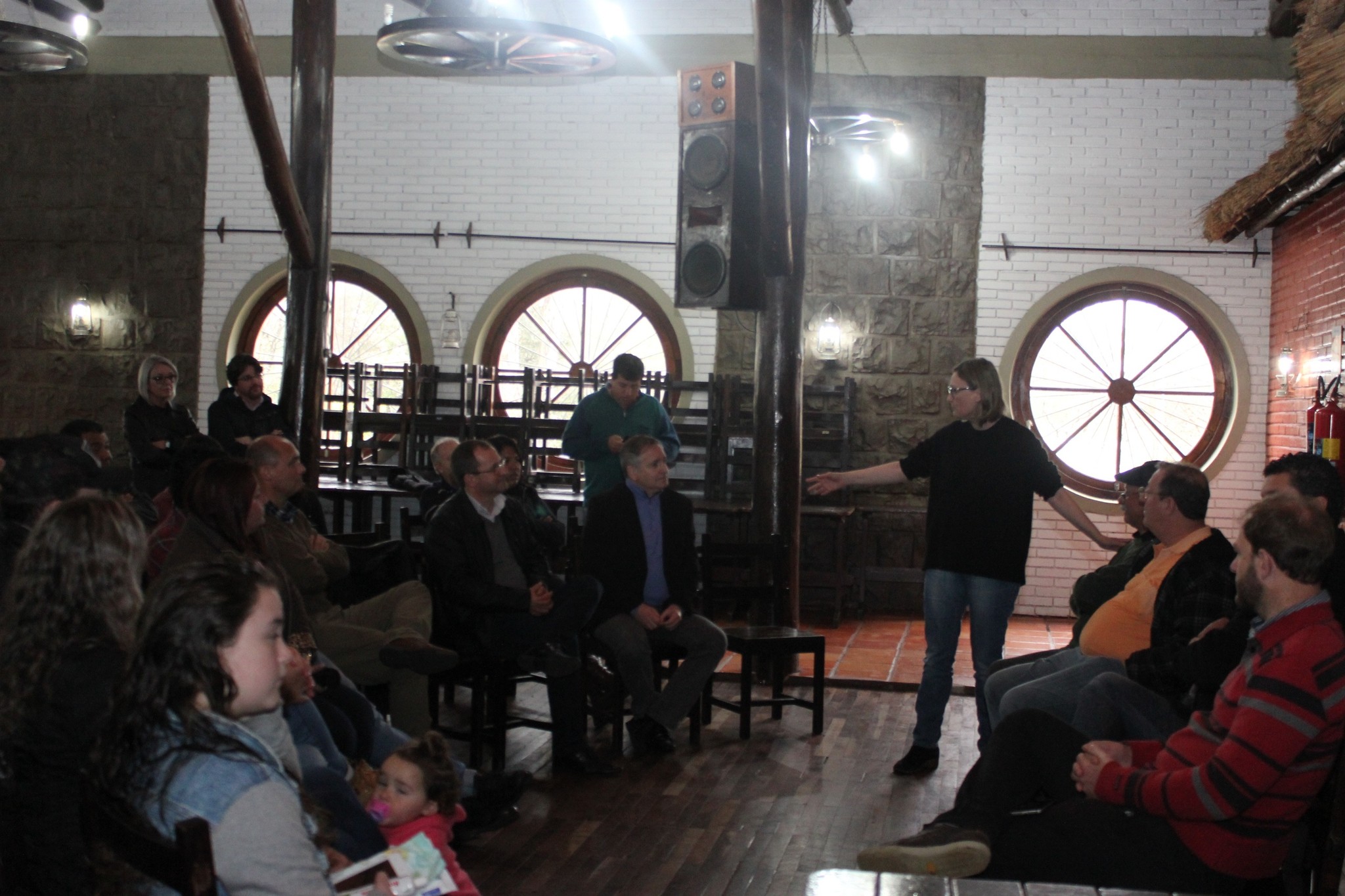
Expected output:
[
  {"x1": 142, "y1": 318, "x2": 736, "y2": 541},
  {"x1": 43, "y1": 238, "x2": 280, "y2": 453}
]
[{"x1": 1115, "y1": 460, "x2": 1163, "y2": 486}]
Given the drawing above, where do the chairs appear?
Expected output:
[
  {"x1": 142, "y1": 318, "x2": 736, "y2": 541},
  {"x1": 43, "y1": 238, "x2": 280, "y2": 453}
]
[
  {"x1": 702, "y1": 524, "x2": 828, "y2": 739},
  {"x1": 404, "y1": 500, "x2": 591, "y2": 777},
  {"x1": 314, "y1": 344, "x2": 859, "y2": 496},
  {"x1": 71, "y1": 772, "x2": 224, "y2": 896},
  {"x1": 568, "y1": 514, "x2": 709, "y2": 757}
]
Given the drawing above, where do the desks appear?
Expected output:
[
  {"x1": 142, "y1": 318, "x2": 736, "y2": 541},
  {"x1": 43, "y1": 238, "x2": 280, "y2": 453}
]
[{"x1": 319, "y1": 473, "x2": 858, "y2": 629}]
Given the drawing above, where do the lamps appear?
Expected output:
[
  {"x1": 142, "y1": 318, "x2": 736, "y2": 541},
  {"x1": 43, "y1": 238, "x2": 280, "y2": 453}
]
[
  {"x1": 810, "y1": 297, "x2": 844, "y2": 363},
  {"x1": 64, "y1": 276, "x2": 97, "y2": 350},
  {"x1": 1273, "y1": 338, "x2": 1294, "y2": 399}
]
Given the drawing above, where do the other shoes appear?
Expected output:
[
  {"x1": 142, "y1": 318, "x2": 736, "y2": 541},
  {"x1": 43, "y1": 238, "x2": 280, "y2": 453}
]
[
  {"x1": 626, "y1": 715, "x2": 678, "y2": 763},
  {"x1": 508, "y1": 640, "x2": 581, "y2": 678},
  {"x1": 549, "y1": 756, "x2": 624, "y2": 778},
  {"x1": 854, "y1": 820, "x2": 991, "y2": 879},
  {"x1": 893, "y1": 747, "x2": 941, "y2": 773}
]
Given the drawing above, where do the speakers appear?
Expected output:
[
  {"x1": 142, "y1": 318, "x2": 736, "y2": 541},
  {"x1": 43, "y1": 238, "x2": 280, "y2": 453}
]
[{"x1": 673, "y1": 117, "x2": 761, "y2": 310}]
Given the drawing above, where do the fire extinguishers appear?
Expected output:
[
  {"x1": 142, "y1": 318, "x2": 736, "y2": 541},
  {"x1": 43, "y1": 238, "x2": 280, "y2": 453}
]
[
  {"x1": 1306, "y1": 376, "x2": 1325, "y2": 453},
  {"x1": 1312, "y1": 374, "x2": 1345, "y2": 477}
]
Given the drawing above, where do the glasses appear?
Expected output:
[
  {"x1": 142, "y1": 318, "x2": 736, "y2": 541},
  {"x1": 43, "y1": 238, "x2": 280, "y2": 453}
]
[
  {"x1": 946, "y1": 384, "x2": 984, "y2": 395},
  {"x1": 147, "y1": 373, "x2": 178, "y2": 385},
  {"x1": 1139, "y1": 486, "x2": 1168, "y2": 500},
  {"x1": 238, "y1": 372, "x2": 265, "y2": 383},
  {"x1": 469, "y1": 459, "x2": 507, "y2": 474}
]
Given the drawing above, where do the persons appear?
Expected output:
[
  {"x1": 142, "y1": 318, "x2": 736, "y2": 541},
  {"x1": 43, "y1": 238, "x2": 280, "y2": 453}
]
[
  {"x1": 805, "y1": 359, "x2": 1134, "y2": 775},
  {"x1": 0, "y1": 352, "x2": 729, "y2": 896},
  {"x1": 857, "y1": 451, "x2": 1345, "y2": 896}
]
